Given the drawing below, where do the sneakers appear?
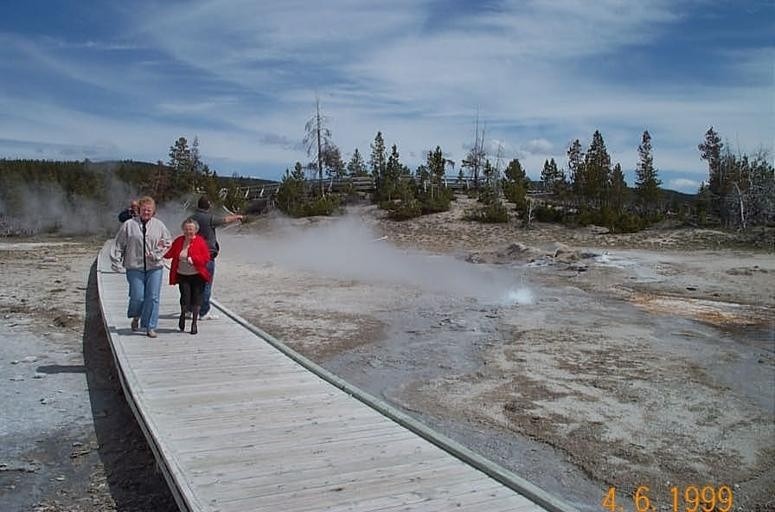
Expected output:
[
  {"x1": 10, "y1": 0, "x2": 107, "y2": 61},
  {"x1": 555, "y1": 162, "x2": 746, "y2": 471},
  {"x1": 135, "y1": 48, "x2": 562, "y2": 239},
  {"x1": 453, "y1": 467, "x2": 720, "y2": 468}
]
[
  {"x1": 147, "y1": 328, "x2": 157, "y2": 338},
  {"x1": 131, "y1": 319, "x2": 139, "y2": 331},
  {"x1": 179, "y1": 317, "x2": 197, "y2": 334},
  {"x1": 199, "y1": 304, "x2": 212, "y2": 320}
]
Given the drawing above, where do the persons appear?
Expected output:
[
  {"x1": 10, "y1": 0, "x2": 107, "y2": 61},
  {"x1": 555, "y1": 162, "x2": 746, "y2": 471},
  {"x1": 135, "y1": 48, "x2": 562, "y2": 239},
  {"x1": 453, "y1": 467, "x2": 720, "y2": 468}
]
[
  {"x1": 118, "y1": 199, "x2": 140, "y2": 222},
  {"x1": 185, "y1": 195, "x2": 250, "y2": 320},
  {"x1": 109, "y1": 196, "x2": 172, "y2": 338},
  {"x1": 163, "y1": 218, "x2": 212, "y2": 335}
]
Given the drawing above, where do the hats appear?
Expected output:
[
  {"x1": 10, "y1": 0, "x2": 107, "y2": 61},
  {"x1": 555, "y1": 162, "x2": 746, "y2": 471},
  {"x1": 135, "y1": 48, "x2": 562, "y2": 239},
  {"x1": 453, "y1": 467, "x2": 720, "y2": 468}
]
[{"x1": 198, "y1": 197, "x2": 210, "y2": 209}]
